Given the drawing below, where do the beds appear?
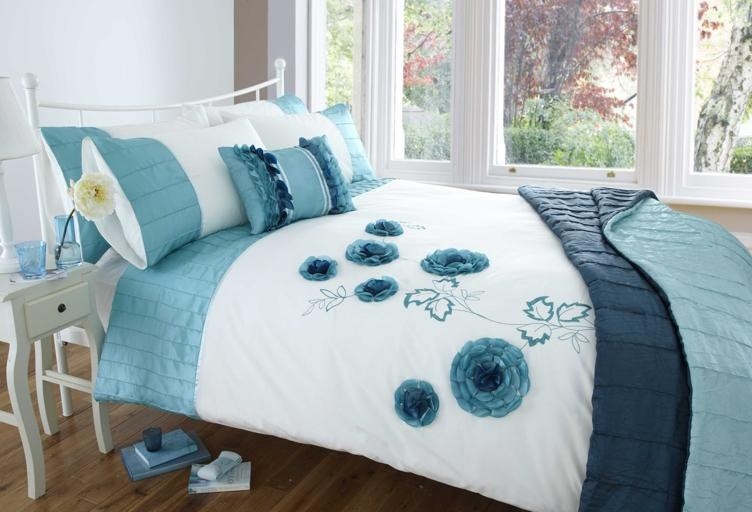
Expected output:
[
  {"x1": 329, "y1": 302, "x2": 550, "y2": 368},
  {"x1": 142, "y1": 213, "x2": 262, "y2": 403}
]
[{"x1": 22, "y1": 57, "x2": 752, "y2": 512}]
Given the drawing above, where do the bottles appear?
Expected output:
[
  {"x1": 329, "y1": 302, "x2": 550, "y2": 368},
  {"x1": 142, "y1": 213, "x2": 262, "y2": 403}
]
[{"x1": 54, "y1": 214, "x2": 82, "y2": 266}]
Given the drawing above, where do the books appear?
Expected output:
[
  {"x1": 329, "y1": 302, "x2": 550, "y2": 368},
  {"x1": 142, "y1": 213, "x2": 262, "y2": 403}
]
[
  {"x1": 118, "y1": 426, "x2": 212, "y2": 482},
  {"x1": 185, "y1": 460, "x2": 253, "y2": 496},
  {"x1": 133, "y1": 427, "x2": 197, "y2": 469}
]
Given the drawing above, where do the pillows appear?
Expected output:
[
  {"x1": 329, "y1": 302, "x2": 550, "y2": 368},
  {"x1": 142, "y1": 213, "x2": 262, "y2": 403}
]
[
  {"x1": 183, "y1": 93, "x2": 310, "y2": 127},
  {"x1": 36, "y1": 105, "x2": 210, "y2": 263},
  {"x1": 216, "y1": 136, "x2": 357, "y2": 234},
  {"x1": 83, "y1": 118, "x2": 268, "y2": 271},
  {"x1": 218, "y1": 102, "x2": 375, "y2": 185}
]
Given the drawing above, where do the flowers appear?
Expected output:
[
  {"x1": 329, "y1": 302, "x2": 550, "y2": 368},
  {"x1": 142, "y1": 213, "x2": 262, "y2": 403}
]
[{"x1": 56, "y1": 173, "x2": 118, "y2": 260}]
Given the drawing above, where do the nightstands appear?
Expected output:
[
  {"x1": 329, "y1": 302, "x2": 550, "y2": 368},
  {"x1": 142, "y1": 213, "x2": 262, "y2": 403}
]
[{"x1": 1, "y1": 268, "x2": 114, "y2": 499}]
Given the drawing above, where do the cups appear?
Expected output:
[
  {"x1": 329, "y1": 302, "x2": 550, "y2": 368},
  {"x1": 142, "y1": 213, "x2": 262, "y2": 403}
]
[
  {"x1": 15, "y1": 239, "x2": 47, "y2": 280},
  {"x1": 142, "y1": 426, "x2": 162, "y2": 451}
]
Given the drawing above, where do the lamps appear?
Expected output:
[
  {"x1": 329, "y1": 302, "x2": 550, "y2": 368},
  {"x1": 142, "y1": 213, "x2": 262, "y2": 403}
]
[{"x1": 0, "y1": 77, "x2": 41, "y2": 272}]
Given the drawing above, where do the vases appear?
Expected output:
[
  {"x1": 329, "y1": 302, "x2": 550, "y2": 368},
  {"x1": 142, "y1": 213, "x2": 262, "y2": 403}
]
[{"x1": 55, "y1": 216, "x2": 79, "y2": 268}]
